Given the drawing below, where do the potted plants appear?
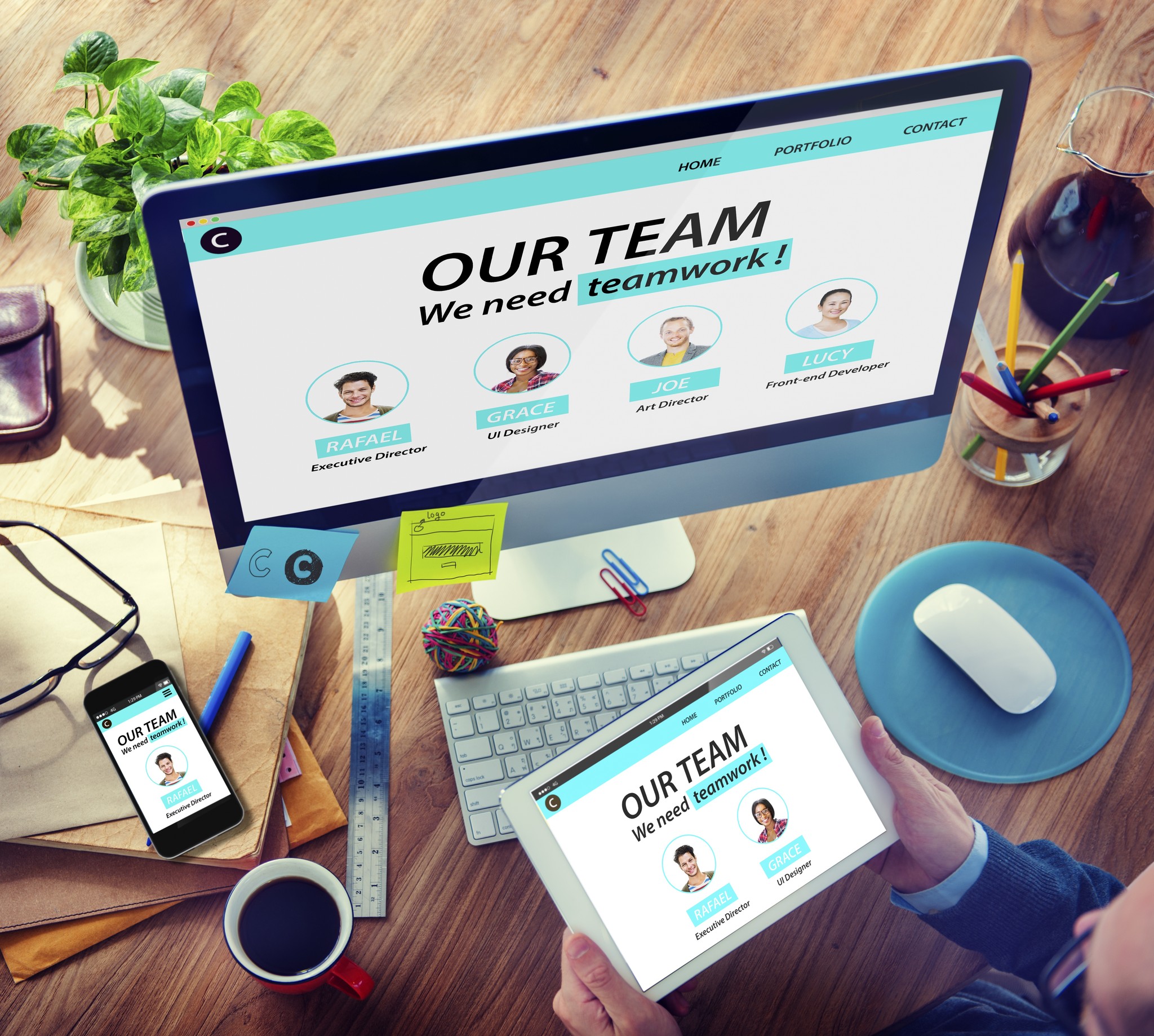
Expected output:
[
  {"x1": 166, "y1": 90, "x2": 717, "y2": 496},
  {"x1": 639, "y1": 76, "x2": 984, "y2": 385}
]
[{"x1": 1, "y1": 30, "x2": 338, "y2": 350}]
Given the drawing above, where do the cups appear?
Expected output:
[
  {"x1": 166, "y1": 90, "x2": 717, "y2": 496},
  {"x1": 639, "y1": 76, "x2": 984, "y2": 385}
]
[
  {"x1": 954, "y1": 342, "x2": 1090, "y2": 487},
  {"x1": 221, "y1": 856, "x2": 376, "y2": 999}
]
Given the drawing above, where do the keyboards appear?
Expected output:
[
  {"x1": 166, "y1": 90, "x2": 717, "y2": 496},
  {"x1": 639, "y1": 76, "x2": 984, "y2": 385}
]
[{"x1": 432, "y1": 610, "x2": 810, "y2": 852}]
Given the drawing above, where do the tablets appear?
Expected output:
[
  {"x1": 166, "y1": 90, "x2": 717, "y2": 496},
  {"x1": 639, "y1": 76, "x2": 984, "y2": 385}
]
[{"x1": 494, "y1": 610, "x2": 903, "y2": 1005}]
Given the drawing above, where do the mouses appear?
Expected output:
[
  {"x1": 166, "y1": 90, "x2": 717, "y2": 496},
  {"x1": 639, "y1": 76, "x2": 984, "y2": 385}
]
[{"x1": 910, "y1": 581, "x2": 1058, "y2": 715}]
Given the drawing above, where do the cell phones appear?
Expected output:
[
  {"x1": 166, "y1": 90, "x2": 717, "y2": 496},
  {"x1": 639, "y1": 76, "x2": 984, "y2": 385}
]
[{"x1": 81, "y1": 656, "x2": 248, "y2": 860}]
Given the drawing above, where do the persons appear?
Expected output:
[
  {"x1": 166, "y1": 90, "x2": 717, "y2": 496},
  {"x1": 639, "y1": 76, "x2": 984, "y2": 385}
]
[
  {"x1": 488, "y1": 343, "x2": 560, "y2": 393},
  {"x1": 322, "y1": 370, "x2": 394, "y2": 425},
  {"x1": 636, "y1": 315, "x2": 710, "y2": 366},
  {"x1": 795, "y1": 284, "x2": 863, "y2": 339},
  {"x1": 674, "y1": 846, "x2": 718, "y2": 893},
  {"x1": 551, "y1": 720, "x2": 1154, "y2": 1036},
  {"x1": 155, "y1": 752, "x2": 185, "y2": 786},
  {"x1": 753, "y1": 798, "x2": 788, "y2": 841}
]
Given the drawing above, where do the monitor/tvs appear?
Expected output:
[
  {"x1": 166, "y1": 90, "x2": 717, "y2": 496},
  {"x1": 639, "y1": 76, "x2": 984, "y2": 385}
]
[{"x1": 134, "y1": 53, "x2": 1032, "y2": 628}]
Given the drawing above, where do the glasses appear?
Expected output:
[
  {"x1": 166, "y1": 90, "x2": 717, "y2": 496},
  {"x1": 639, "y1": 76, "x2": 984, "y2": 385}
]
[
  {"x1": 509, "y1": 356, "x2": 540, "y2": 365},
  {"x1": 1039, "y1": 923, "x2": 1094, "y2": 1036},
  {"x1": 754, "y1": 808, "x2": 769, "y2": 818}
]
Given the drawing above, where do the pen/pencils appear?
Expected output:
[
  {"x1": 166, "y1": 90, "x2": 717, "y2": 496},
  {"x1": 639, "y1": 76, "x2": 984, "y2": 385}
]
[
  {"x1": 1019, "y1": 367, "x2": 1130, "y2": 402},
  {"x1": 1031, "y1": 400, "x2": 1061, "y2": 422},
  {"x1": 143, "y1": 630, "x2": 252, "y2": 855},
  {"x1": 958, "y1": 271, "x2": 1120, "y2": 456},
  {"x1": 997, "y1": 360, "x2": 1027, "y2": 410},
  {"x1": 971, "y1": 308, "x2": 1043, "y2": 481},
  {"x1": 993, "y1": 249, "x2": 1025, "y2": 481}
]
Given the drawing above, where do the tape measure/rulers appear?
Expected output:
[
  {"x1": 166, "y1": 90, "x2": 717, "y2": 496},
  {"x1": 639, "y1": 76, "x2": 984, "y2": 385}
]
[{"x1": 337, "y1": 572, "x2": 392, "y2": 920}]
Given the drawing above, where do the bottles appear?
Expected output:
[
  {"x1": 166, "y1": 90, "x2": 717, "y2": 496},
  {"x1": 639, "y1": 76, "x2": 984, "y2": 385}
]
[{"x1": 1007, "y1": 85, "x2": 1154, "y2": 341}]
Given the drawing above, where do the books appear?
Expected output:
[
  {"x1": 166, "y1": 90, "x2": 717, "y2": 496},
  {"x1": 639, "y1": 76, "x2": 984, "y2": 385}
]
[
  {"x1": 0, "y1": 471, "x2": 320, "y2": 870},
  {"x1": 0, "y1": 477, "x2": 291, "y2": 942}
]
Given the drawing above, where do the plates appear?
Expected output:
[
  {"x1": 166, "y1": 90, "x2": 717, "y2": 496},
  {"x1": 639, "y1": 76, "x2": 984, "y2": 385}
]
[{"x1": 75, "y1": 210, "x2": 174, "y2": 353}]
